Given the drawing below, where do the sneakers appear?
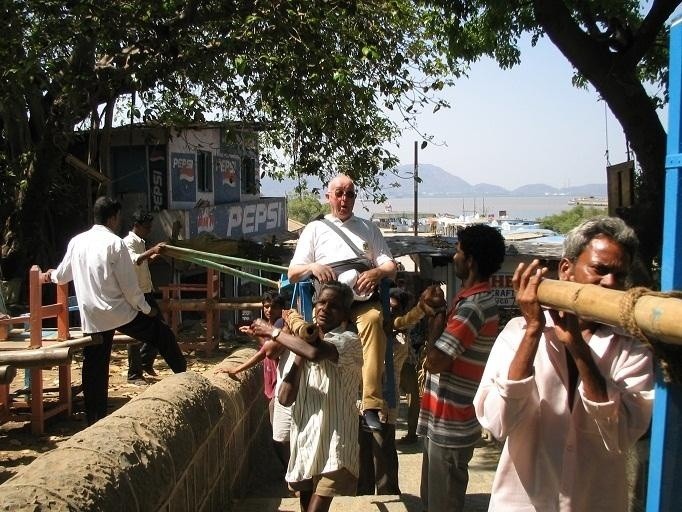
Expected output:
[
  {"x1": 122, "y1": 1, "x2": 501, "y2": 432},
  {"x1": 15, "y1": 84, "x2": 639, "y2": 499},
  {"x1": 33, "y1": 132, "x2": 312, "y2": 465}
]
[{"x1": 396, "y1": 433, "x2": 419, "y2": 444}]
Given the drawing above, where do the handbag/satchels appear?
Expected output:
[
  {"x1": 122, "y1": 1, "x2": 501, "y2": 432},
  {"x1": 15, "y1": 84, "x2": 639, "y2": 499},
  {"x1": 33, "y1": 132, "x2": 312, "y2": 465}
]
[{"x1": 311, "y1": 257, "x2": 381, "y2": 306}]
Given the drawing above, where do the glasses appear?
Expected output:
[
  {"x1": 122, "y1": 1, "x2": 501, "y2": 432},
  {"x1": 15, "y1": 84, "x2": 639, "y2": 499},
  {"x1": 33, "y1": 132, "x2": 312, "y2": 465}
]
[{"x1": 327, "y1": 189, "x2": 356, "y2": 199}]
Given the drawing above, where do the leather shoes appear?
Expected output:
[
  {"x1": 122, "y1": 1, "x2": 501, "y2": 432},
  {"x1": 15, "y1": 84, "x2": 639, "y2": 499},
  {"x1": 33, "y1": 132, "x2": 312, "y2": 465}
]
[
  {"x1": 363, "y1": 409, "x2": 384, "y2": 431},
  {"x1": 126, "y1": 376, "x2": 148, "y2": 385},
  {"x1": 141, "y1": 363, "x2": 158, "y2": 377}
]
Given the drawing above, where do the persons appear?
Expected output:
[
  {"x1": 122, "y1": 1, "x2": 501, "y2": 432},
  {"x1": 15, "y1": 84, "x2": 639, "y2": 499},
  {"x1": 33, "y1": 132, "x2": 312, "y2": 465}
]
[
  {"x1": 470, "y1": 216, "x2": 657, "y2": 512},
  {"x1": 39, "y1": 195, "x2": 187, "y2": 425},
  {"x1": 121, "y1": 210, "x2": 168, "y2": 385}
]
